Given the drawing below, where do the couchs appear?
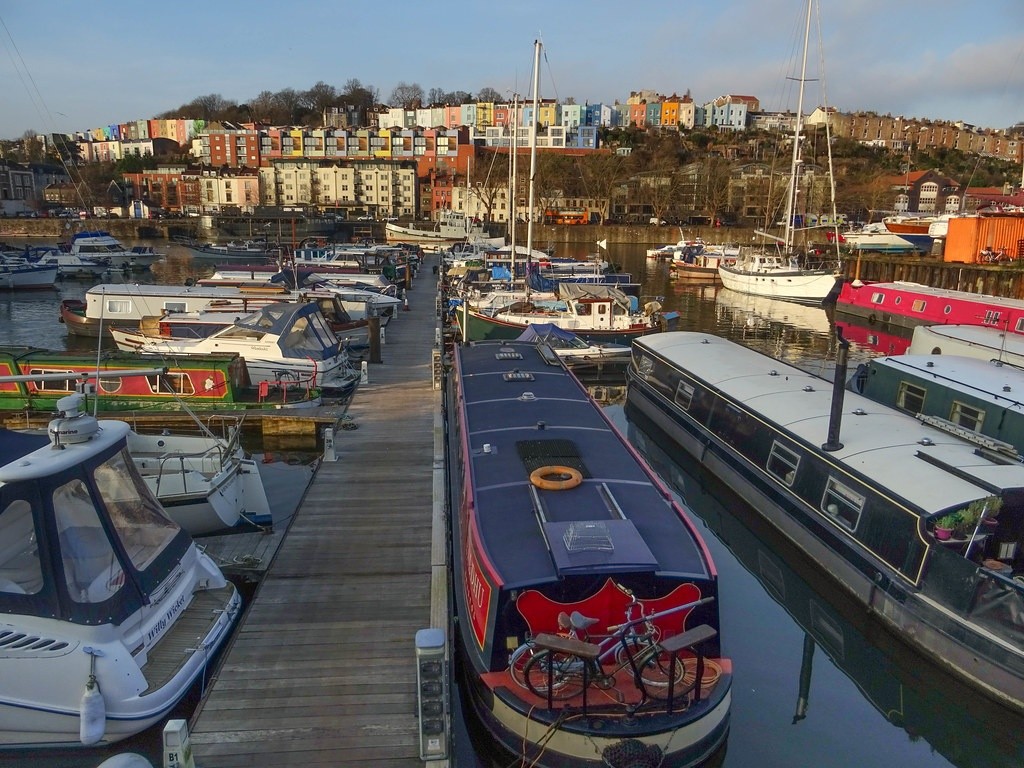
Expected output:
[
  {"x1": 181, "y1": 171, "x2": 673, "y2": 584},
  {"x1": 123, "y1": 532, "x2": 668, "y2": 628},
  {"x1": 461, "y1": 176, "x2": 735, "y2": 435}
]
[{"x1": 0, "y1": 521, "x2": 176, "y2": 606}]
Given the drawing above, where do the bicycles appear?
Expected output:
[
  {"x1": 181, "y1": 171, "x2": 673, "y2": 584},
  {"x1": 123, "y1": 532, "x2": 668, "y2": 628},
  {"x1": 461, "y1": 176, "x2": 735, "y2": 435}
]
[
  {"x1": 503, "y1": 582, "x2": 684, "y2": 693},
  {"x1": 524, "y1": 600, "x2": 686, "y2": 701}
]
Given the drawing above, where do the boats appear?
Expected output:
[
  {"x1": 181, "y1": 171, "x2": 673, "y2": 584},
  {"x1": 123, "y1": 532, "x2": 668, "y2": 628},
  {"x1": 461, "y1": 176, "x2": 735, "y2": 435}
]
[
  {"x1": 621, "y1": 165, "x2": 1024, "y2": 718},
  {"x1": 0, "y1": 148, "x2": 424, "y2": 546},
  {"x1": 1, "y1": 391, "x2": 242, "y2": 754},
  {"x1": 443, "y1": 334, "x2": 735, "y2": 768}
]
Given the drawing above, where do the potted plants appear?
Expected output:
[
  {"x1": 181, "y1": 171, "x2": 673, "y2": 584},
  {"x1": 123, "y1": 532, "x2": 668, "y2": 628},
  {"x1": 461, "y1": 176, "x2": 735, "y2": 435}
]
[
  {"x1": 984, "y1": 495, "x2": 1001, "y2": 533},
  {"x1": 934, "y1": 514, "x2": 955, "y2": 541},
  {"x1": 967, "y1": 502, "x2": 982, "y2": 535},
  {"x1": 952, "y1": 508, "x2": 974, "y2": 540}
]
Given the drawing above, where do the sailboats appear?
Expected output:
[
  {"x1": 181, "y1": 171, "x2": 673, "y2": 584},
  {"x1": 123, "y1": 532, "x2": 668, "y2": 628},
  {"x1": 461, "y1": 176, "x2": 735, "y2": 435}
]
[
  {"x1": 716, "y1": 1, "x2": 849, "y2": 306},
  {"x1": 381, "y1": 33, "x2": 680, "y2": 381}
]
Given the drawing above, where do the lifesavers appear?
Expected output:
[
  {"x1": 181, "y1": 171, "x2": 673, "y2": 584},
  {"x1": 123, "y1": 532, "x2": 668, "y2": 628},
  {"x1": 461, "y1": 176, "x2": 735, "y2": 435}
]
[{"x1": 529, "y1": 464, "x2": 582, "y2": 491}]
